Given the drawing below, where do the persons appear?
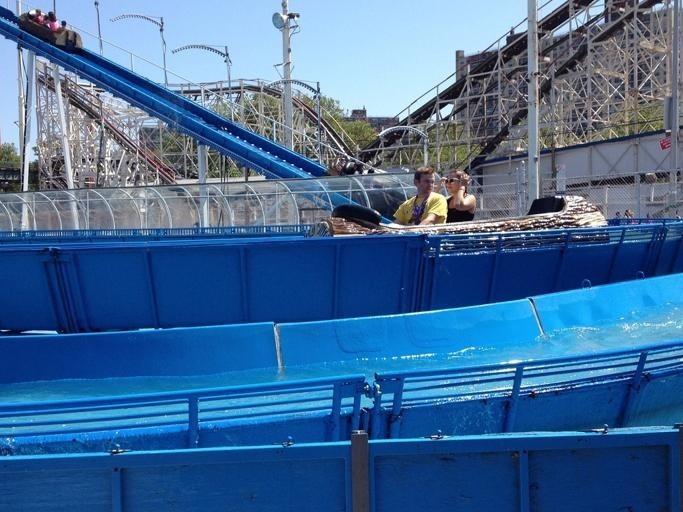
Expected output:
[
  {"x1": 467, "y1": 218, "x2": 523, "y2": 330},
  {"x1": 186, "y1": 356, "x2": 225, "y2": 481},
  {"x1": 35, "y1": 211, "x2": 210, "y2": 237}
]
[
  {"x1": 434, "y1": 168, "x2": 477, "y2": 222},
  {"x1": 331, "y1": 156, "x2": 375, "y2": 188},
  {"x1": 616, "y1": 208, "x2": 682, "y2": 225},
  {"x1": 29, "y1": 9, "x2": 66, "y2": 33},
  {"x1": 393, "y1": 166, "x2": 448, "y2": 226}
]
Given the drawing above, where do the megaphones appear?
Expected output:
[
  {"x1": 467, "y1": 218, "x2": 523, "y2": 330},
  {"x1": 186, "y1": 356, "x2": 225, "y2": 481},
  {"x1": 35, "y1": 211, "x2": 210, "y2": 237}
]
[{"x1": 273, "y1": 12, "x2": 290, "y2": 29}]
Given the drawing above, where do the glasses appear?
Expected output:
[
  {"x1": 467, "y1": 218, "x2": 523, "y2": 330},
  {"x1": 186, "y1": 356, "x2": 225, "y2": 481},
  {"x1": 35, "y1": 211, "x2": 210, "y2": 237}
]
[{"x1": 445, "y1": 178, "x2": 460, "y2": 183}]
